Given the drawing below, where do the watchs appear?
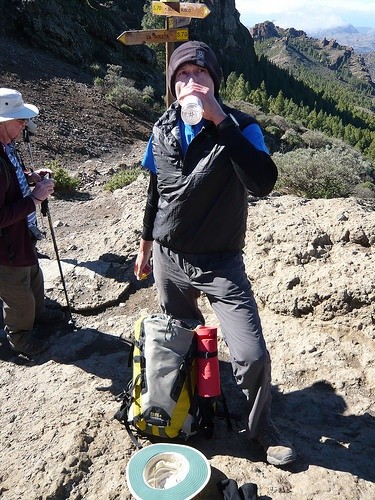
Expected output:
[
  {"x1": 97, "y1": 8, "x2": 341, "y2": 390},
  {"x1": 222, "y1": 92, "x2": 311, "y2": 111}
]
[{"x1": 215, "y1": 113, "x2": 240, "y2": 134}]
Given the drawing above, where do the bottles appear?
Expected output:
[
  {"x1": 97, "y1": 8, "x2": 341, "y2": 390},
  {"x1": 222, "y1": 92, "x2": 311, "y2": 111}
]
[{"x1": 180, "y1": 95, "x2": 204, "y2": 125}]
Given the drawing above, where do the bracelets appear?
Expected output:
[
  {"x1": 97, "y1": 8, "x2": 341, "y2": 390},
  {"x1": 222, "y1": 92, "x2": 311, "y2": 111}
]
[{"x1": 29, "y1": 192, "x2": 47, "y2": 202}]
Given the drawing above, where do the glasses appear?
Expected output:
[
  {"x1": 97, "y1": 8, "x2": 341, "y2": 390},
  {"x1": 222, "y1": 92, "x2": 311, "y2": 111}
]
[{"x1": 16, "y1": 118, "x2": 30, "y2": 123}]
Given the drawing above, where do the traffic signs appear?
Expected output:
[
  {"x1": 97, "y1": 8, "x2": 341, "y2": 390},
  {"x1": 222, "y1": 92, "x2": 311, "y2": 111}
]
[
  {"x1": 117, "y1": 27, "x2": 189, "y2": 47},
  {"x1": 152, "y1": 1, "x2": 210, "y2": 18}
]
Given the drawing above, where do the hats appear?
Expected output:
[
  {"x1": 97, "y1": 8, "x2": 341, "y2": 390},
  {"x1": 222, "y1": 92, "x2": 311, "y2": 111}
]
[
  {"x1": 0, "y1": 87, "x2": 40, "y2": 123},
  {"x1": 125, "y1": 443, "x2": 212, "y2": 500},
  {"x1": 168, "y1": 40, "x2": 221, "y2": 99}
]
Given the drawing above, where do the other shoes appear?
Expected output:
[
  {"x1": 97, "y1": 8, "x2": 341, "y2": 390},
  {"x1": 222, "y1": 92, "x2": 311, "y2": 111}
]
[
  {"x1": 9, "y1": 334, "x2": 50, "y2": 356},
  {"x1": 35, "y1": 307, "x2": 66, "y2": 324},
  {"x1": 254, "y1": 423, "x2": 298, "y2": 466}
]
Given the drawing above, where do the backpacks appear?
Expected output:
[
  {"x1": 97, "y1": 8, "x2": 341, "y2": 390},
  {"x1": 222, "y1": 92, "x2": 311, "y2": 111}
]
[{"x1": 113, "y1": 312, "x2": 234, "y2": 449}]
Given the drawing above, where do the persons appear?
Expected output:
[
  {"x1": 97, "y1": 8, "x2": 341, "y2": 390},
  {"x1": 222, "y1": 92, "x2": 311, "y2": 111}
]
[
  {"x1": 0, "y1": 87, "x2": 66, "y2": 357},
  {"x1": 133, "y1": 41, "x2": 299, "y2": 466}
]
